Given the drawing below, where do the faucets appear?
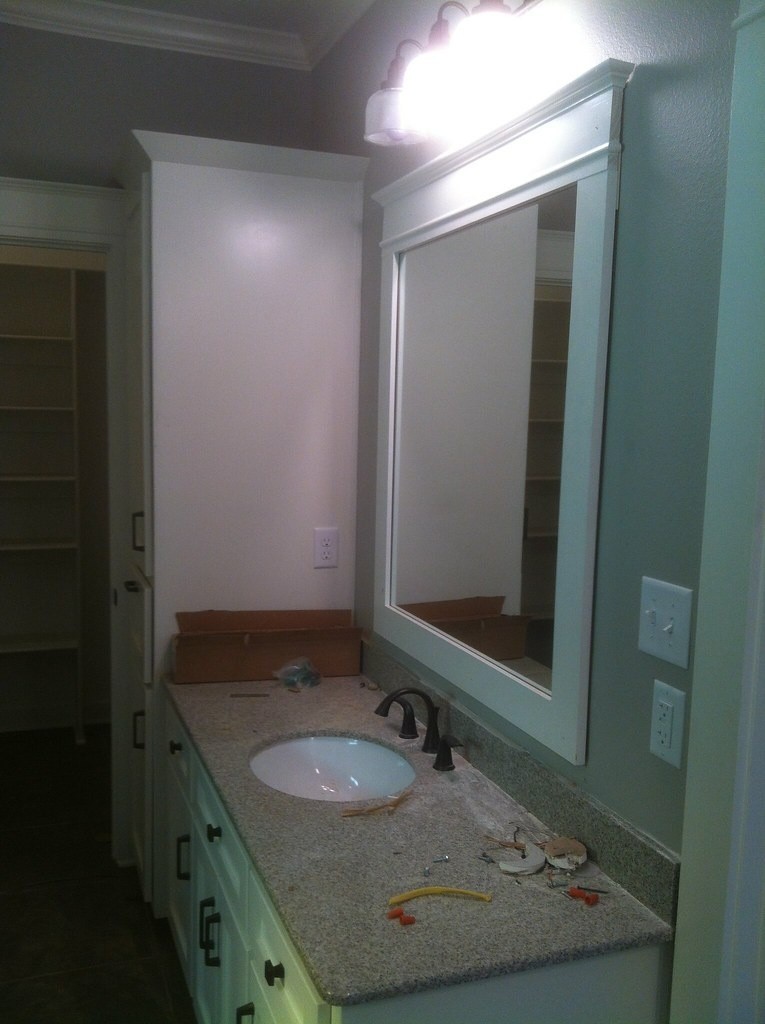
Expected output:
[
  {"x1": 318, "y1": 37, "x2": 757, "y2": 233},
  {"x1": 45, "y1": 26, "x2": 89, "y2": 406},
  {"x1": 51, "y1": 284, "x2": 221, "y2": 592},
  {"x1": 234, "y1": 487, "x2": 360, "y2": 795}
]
[{"x1": 375, "y1": 687, "x2": 441, "y2": 755}]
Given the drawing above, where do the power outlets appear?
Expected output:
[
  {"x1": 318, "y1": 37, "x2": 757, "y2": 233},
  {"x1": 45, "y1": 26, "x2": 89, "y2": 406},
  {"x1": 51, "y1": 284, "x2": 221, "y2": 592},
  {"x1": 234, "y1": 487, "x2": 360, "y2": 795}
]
[
  {"x1": 648, "y1": 679, "x2": 687, "y2": 769},
  {"x1": 312, "y1": 527, "x2": 339, "y2": 569}
]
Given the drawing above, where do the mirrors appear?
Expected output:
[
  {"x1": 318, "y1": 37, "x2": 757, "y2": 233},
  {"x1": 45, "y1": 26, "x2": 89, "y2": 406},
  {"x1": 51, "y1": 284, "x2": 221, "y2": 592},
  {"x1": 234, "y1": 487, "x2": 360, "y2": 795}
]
[{"x1": 357, "y1": 55, "x2": 636, "y2": 770}]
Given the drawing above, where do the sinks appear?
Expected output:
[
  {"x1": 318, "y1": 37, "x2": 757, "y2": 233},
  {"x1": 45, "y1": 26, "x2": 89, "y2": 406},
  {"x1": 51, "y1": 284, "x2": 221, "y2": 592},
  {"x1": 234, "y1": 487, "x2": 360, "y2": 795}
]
[{"x1": 245, "y1": 728, "x2": 418, "y2": 803}]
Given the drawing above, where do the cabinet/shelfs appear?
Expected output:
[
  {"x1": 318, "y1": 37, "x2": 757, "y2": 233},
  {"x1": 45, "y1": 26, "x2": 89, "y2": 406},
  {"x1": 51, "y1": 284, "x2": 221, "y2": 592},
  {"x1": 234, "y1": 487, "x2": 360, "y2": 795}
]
[
  {"x1": 521, "y1": 280, "x2": 572, "y2": 663},
  {"x1": 0, "y1": 241, "x2": 109, "y2": 750},
  {"x1": 110, "y1": 128, "x2": 365, "y2": 923},
  {"x1": 158, "y1": 673, "x2": 678, "y2": 1024}
]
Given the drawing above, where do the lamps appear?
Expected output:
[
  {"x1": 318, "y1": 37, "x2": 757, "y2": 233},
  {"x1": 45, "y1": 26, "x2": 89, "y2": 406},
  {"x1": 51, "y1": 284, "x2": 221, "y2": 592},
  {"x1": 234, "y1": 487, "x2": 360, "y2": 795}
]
[{"x1": 364, "y1": 0, "x2": 541, "y2": 150}]
